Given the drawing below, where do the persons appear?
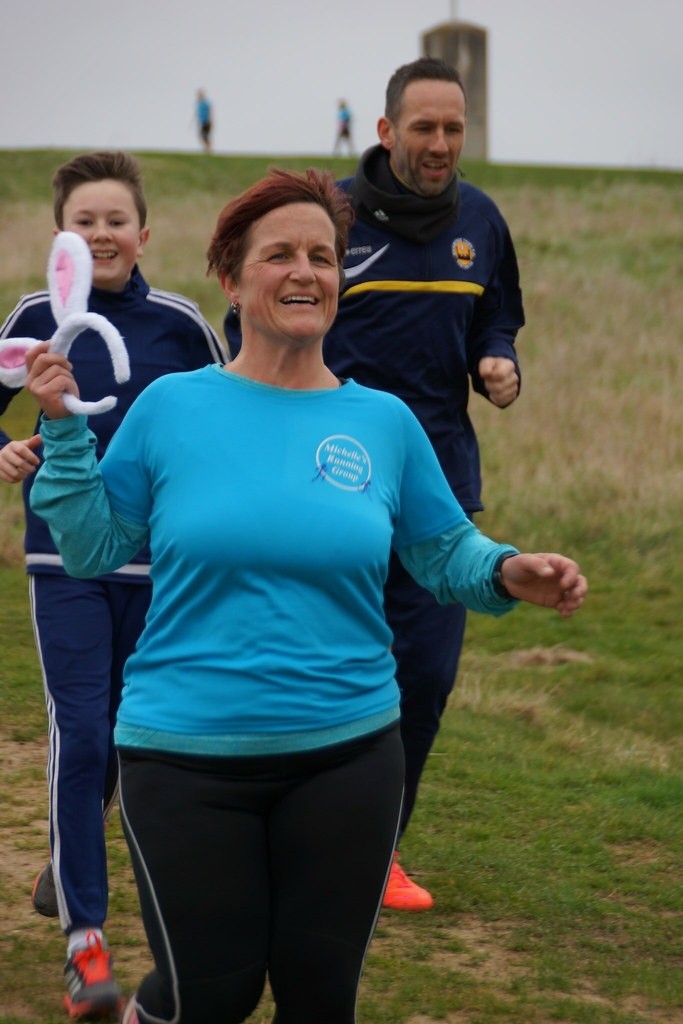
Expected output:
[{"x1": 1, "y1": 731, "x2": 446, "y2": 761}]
[
  {"x1": 223, "y1": 59, "x2": 523, "y2": 911},
  {"x1": 24, "y1": 165, "x2": 590, "y2": 1024},
  {"x1": 0, "y1": 149, "x2": 222, "y2": 1024},
  {"x1": 191, "y1": 87, "x2": 213, "y2": 152},
  {"x1": 332, "y1": 98, "x2": 356, "y2": 157}
]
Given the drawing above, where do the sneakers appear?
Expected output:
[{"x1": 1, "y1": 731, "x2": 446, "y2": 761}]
[
  {"x1": 60, "y1": 931, "x2": 127, "y2": 1022},
  {"x1": 31, "y1": 854, "x2": 63, "y2": 917},
  {"x1": 382, "y1": 851, "x2": 434, "y2": 910}
]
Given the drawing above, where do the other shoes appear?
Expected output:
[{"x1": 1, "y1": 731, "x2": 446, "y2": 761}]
[{"x1": 123, "y1": 993, "x2": 139, "y2": 1024}]
